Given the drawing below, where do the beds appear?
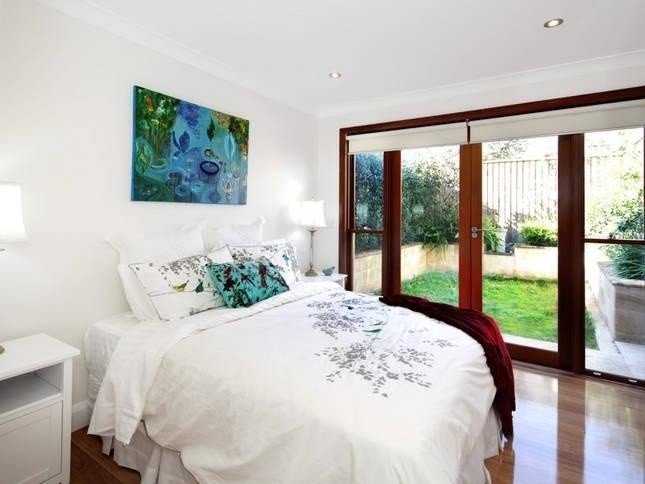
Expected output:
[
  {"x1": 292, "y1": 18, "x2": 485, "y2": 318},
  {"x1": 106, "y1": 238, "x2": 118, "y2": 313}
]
[{"x1": 84, "y1": 280, "x2": 498, "y2": 484}]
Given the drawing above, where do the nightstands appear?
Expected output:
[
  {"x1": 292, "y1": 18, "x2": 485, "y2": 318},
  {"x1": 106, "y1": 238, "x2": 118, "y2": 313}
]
[
  {"x1": 0, "y1": 333, "x2": 82, "y2": 484},
  {"x1": 301, "y1": 271, "x2": 348, "y2": 289}
]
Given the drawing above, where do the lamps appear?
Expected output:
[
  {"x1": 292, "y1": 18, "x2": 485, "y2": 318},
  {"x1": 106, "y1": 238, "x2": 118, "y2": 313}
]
[
  {"x1": 0, "y1": 182, "x2": 30, "y2": 354},
  {"x1": 300, "y1": 196, "x2": 327, "y2": 277}
]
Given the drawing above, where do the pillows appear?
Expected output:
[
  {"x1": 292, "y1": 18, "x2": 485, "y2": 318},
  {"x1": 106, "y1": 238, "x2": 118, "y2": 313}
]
[{"x1": 105, "y1": 215, "x2": 303, "y2": 322}]
